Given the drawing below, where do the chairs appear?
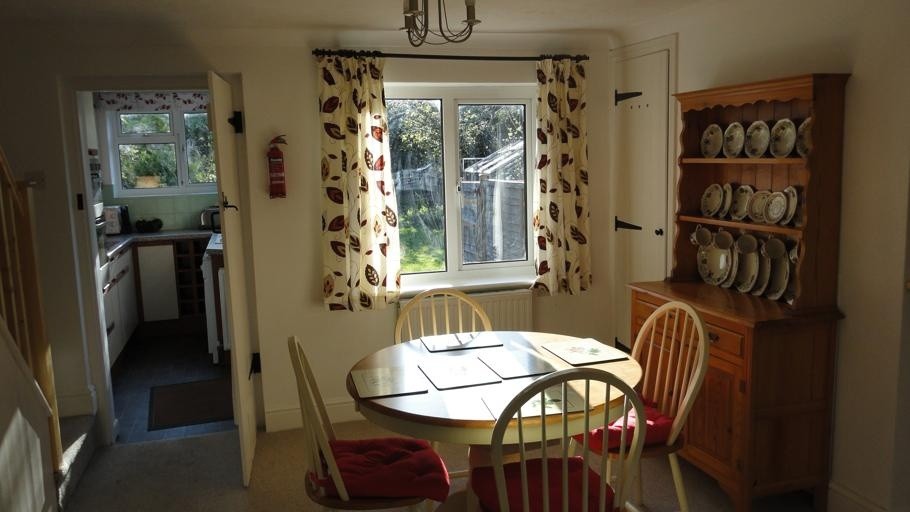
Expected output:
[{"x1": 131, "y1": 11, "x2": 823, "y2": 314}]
[
  {"x1": 564, "y1": 302, "x2": 711, "y2": 511},
  {"x1": 286, "y1": 334, "x2": 450, "y2": 512},
  {"x1": 464, "y1": 366, "x2": 649, "y2": 512},
  {"x1": 394, "y1": 287, "x2": 495, "y2": 471}
]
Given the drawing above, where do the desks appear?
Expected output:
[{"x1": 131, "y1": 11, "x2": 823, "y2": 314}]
[{"x1": 346, "y1": 330, "x2": 642, "y2": 510}]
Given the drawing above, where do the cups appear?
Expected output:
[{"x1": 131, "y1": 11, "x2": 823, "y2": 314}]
[{"x1": 689, "y1": 223, "x2": 800, "y2": 264}]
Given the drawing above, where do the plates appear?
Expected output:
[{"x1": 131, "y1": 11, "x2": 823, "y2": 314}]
[
  {"x1": 700, "y1": 182, "x2": 803, "y2": 227},
  {"x1": 699, "y1": 117, "x2": 812, "y2": 159},
  {"x1": 695, "y1": 234, "x2": 796, "y2": 305}
]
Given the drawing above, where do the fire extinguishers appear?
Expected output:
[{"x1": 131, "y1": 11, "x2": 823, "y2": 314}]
[{"x1": 267, "y1": 134, "x2": 288, "y2": 199}]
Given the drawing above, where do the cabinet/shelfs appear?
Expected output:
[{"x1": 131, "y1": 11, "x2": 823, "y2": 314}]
[
  {"x1": 134, "y1": 239, "x2": 211, "y2": 326},
  {"x1": 626, "y1": 72, "x2": 850, "y2": 511},
  {"x1": 98, "y1": 240, "x2": 138, "y2": 369},
  {"x1": 202, "y1": 238, "x2": 232, "y2": 364}
]
